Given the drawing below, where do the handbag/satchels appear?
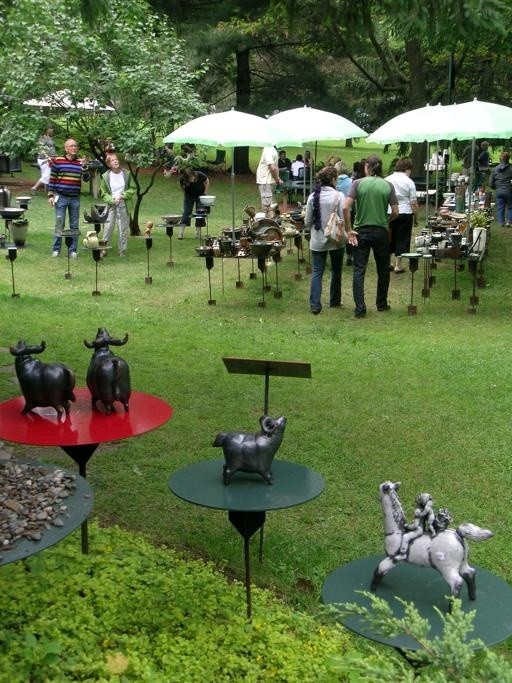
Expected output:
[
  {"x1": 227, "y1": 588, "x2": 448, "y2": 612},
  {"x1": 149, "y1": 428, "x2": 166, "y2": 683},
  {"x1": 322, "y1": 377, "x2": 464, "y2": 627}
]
[
  {"x1": 324, "y1": 213, "x2": 344, "y2": 243},
  {"x1": 479, "y1": 167, "x2": 490, "y2": 176}
]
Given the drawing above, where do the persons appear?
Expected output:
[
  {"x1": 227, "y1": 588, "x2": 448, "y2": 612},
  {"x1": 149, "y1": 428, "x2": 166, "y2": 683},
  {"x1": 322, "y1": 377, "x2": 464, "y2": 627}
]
[
  {"x1": 162, "y1": 142, "x2": 210, "y2": 240},
  {"x1": 30, "y1": 127, "x2": 137, "y2": 262},
  {"x1": 256, "y1": 145, "x2": 419, "y2": 319},
  {"x1": 393, "y1": 493, "x2": 436, "y2": 562}
]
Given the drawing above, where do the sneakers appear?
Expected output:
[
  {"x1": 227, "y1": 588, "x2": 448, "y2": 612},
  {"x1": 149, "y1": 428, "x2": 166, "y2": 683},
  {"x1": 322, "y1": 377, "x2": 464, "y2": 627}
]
[
  {"x1": 506, "y1": 223, "x2": 510, "y2": 226},
  {"x1": 312, "y1": 310, "x2": 319, "y2": 313},
  {"x1": 195, "y1": 232, "x2": 200, "y2": 239},
  {"x1": 51, "y1": 252, "x2": 59, "y2": 257},
  {"x1": 378, "y1": 305, "x2": 390, "y2": 311},
  {"x1": 340, "y1": 302, "x2": 342, "y2": 306},
  {"x1": 395, "y1": 267, "x2": 404, "y2": 273},
  {"x1": 177, "y1": 233, "x2": 184, "y2": 238},
  {"x1": 390, "y1": 264, "x2": 394, "y2": 272},
  {"x1": 69, "y1": 252, "x2": 79, "y2": 259}
]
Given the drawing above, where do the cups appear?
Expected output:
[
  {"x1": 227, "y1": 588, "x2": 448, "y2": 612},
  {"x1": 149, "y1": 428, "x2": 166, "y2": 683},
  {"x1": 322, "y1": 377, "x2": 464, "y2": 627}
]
[
  {"x1": 446, "y1": 226, "x2": 467, "y2": 250},
  {"x1": 423, "y1": 152, "x2": 445, "y2": 170}
]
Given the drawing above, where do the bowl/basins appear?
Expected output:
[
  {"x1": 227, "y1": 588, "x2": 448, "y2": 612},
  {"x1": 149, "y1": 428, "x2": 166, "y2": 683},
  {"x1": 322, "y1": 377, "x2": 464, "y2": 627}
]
[
  {"x1": 199, "y1": 195, "x2": 216, "y2": 206},
  {"x1": 196, "y1": 209, "x2": 304, "y2": 260},
  {"x1": 1, "y1": 207, "x2": 27, "y2": 219},
  {"x1": 15, "y1": 196, "x2": 33, "y2": 201},
  {"x1": 446, "y1": 172, "x2": 471, "y2": 187},
  {"x1": 162, "y1": 214, "x2": 184, "y2": 224}
]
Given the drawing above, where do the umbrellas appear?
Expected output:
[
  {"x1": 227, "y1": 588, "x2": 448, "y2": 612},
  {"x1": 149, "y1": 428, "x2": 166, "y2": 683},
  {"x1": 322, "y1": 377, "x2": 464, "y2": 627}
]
[
  {"x1": 162, "y1": 107, "x2": 266, "y2": 241},
  {"x1": 21, "y1": 89, "x2": 117, "y2": 111},
  {"x1": 366, "y1": 96, "x2": 512, "y2": 237},
  {"x1": 266, "y1": 104, "x2": 368, "y2": 195}
]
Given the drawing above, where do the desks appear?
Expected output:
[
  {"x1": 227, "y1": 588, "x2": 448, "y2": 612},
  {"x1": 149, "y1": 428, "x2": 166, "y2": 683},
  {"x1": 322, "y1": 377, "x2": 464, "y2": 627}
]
[
  {"x1": 320, "y1": 554, "x2": 511, "y2": 683},
  {"x1": 166, "y1": 457, "x2": 326, "y2": 622},
  {"x1": 1, "y1": 186, "x2": 491, "y2": 317},
  {"x1": 0, "y1": 385, "x2": 175, "y2": 552},
  {"x1": 0, "y1": 455, "x2": 97, "y2": 569}
]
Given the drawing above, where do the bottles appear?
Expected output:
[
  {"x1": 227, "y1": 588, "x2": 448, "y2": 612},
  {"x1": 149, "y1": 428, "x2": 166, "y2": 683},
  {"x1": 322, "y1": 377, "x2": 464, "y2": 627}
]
[{"x1": 85, "y1": 230, "x2": 99, "y2": 248}]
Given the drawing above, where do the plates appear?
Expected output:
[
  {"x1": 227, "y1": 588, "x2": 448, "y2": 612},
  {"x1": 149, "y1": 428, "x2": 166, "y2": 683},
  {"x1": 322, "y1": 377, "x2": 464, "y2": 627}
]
[{"x1": 399, "y1": 252, "x2": 422, "y2": 257}]
